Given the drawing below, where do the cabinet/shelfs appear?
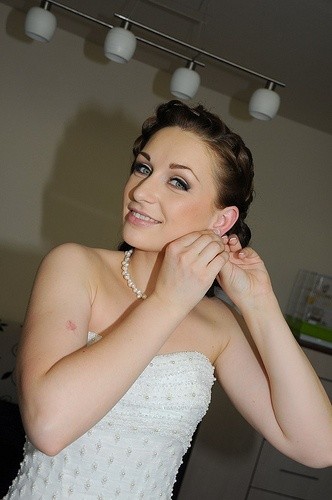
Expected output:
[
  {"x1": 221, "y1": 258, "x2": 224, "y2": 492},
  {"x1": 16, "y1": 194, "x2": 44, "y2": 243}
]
[{"x1": 173, "y1": 315, "x2": 332, "y2": 500}]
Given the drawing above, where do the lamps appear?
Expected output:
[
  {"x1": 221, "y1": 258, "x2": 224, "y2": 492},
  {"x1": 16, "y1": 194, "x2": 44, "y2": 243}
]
[{"x1": 18, "y1": 0, "x2": 286, "y2": 122}]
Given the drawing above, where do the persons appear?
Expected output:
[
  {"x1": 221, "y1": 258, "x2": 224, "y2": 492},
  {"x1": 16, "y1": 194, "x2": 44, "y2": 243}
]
[{"x1": 0, "y1": 98, "x2": 332, "y2": 500}]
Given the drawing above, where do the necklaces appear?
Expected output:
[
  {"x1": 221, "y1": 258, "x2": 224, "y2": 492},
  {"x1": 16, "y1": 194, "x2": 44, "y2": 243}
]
[{"x1": 120, "y1": 247, "x2": 150, "y2": 301}]
